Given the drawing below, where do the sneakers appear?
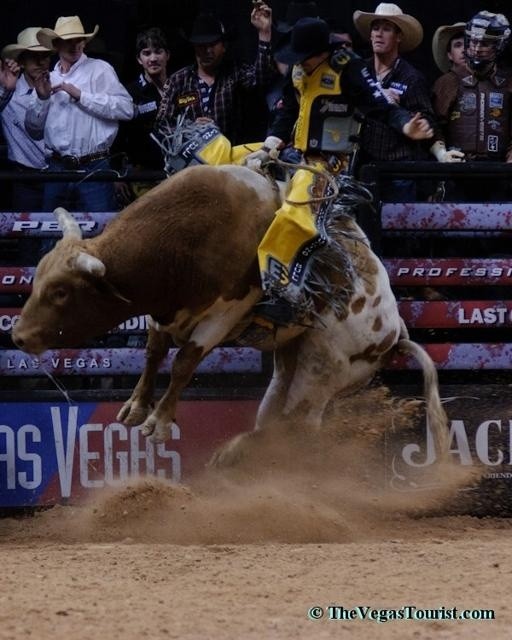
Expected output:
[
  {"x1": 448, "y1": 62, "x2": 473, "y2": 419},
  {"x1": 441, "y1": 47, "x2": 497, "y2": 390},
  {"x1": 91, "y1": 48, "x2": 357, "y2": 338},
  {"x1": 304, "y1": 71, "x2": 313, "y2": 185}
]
[{"x1": 252, "y1": 297, "x2": 292, "y2": 322}]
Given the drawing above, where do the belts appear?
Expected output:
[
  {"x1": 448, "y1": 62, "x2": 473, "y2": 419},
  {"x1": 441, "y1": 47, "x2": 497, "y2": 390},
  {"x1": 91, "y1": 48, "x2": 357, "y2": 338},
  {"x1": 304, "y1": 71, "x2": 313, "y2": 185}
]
[{"x1": 52, "y1": 151, "x2": 107, "y2": 168}]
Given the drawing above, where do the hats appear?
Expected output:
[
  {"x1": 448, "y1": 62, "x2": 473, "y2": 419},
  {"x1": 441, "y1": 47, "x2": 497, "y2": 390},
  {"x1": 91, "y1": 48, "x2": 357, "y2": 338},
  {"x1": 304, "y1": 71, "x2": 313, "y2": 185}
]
[
  {"x1": 353, "y1": 2, "x2": 424, "y2": 51},
  {"x1": 188, "y1": 16, "x2": 227, "y2": 43},
  {"x1": 275, "y1": 16, "x2": 345, "y2": 64},
  {"x1": 36, "y1": 16, "x2": 99, "y2": 50},
  {"x1": 432, "y1": 22, "x2": 467, "y2": 73},
  {"x1": 2, "y1": 27, "x2": 53, "y2": 60}
]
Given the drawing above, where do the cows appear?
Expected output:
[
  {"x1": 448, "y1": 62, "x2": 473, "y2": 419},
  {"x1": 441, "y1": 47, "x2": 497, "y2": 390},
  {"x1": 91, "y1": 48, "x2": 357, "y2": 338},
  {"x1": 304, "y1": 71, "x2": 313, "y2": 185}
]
[{"x1": 8, "y1": 162, "x2": 486, "y2": 486}]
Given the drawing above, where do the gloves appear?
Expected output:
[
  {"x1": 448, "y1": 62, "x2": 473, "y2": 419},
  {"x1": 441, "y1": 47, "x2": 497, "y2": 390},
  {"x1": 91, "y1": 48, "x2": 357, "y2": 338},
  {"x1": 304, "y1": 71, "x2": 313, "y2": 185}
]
[
  {"x1": 244, "y1": 136, "x2": 286, "y2": 168},
  {"x1": 430, "y1": 140, "x2": 464, "y2": 163}
]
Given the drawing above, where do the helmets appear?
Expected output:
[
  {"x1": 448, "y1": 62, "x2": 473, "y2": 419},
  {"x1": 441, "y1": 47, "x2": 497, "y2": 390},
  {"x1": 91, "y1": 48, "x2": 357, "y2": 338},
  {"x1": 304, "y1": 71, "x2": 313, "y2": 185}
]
[{"x1": 463, "y1": 12, "x2": 511, "y2": 75}]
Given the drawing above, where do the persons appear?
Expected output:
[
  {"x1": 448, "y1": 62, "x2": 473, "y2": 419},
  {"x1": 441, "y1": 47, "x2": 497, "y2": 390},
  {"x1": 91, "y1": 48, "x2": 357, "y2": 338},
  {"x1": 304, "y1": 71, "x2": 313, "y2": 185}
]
[{"x1": 0, "y1": 0, "x2": 512, "y2": 325}]
